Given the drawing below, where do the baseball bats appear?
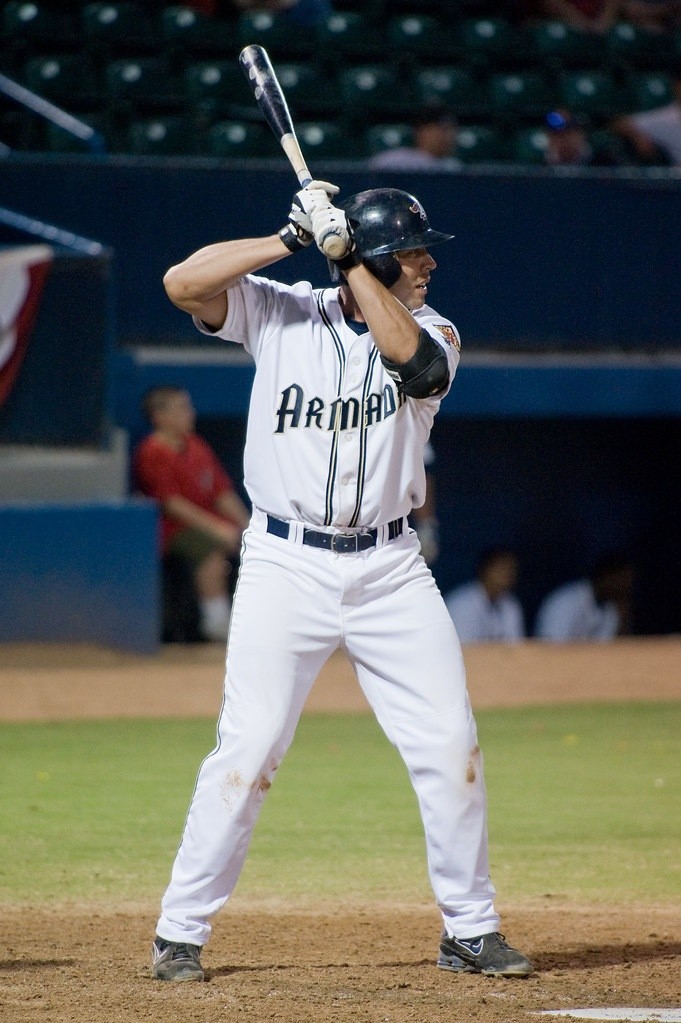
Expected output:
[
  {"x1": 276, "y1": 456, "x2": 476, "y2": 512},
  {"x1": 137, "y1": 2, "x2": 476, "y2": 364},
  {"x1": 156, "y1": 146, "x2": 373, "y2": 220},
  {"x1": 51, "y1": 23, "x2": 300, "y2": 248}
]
[{"x1": 237, "y1": 44, "x2": 347, "y2": 259}]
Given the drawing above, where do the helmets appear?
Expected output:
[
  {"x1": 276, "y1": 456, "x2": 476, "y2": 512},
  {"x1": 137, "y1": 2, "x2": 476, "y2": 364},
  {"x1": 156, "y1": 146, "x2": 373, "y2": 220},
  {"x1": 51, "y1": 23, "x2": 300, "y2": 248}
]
[{"x1": 327, "y1": 189, "x2": 455, "y2": 282}]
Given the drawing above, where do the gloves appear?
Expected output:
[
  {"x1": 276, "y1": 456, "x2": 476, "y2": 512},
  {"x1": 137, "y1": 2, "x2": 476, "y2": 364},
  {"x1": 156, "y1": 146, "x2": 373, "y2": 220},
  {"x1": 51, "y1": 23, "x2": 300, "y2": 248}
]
[
  {"x1": 309, "y1": 206, "x2": 362, "y2": 270},
  {"x1": 278, "y1": 180, "x2": 340, "y2": 254}
]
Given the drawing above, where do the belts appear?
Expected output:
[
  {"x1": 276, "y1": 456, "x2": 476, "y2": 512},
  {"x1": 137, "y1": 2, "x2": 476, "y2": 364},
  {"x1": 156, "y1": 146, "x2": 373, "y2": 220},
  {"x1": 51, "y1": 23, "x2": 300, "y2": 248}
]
[{"x1": 266, "y1": 516, "x2": 403, "y2": 554}]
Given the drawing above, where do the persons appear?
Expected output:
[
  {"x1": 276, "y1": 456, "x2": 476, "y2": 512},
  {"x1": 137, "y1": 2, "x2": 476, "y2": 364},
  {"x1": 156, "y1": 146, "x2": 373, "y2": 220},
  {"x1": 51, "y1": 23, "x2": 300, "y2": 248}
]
[
  {"x1": 530, "y1": 105, "x2": 615, "y2": 166},
  {"x1": 147, "y1": 188, "x2": 535, "y2": 984},
  {"x1": 614, "y1": 83, "x2": 681, "y2": 165},
  {"x1": 412, "y1": 437, "x2": 439, "y2": 563},
  {"x1": 443, "y1": 543, "x2": 527, "y2": 640},
  {"x1": 367, "y1": 113, "x2": 470, "y2": 174},
  {"x1": 537, "y1": 553, "x2": 624, "y2": 641},
  {"x1": 133, "y1": 384, "x2": 251, "y2": 640},
  {"x1": 546, "y1": 0, "x2": 681, "y2": 38}
]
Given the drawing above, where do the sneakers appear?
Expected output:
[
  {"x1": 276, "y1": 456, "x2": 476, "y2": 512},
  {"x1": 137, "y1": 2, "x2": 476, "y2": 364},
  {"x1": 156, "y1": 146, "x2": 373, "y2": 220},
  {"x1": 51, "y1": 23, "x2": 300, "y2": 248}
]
[
  {"x1": 438, "y1": 929, "x2": 530, "y2": 976},
  {"x1": 152, "y1": 936, "x2": 206, "y2": 982}
]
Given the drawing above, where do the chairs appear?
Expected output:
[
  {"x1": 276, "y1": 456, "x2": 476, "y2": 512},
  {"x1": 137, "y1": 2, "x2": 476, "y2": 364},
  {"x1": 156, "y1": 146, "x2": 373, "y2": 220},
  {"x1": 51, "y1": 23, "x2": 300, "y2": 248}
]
[{"x1": 3, "y1": 0, "x2": 679, "y2": 164}]
